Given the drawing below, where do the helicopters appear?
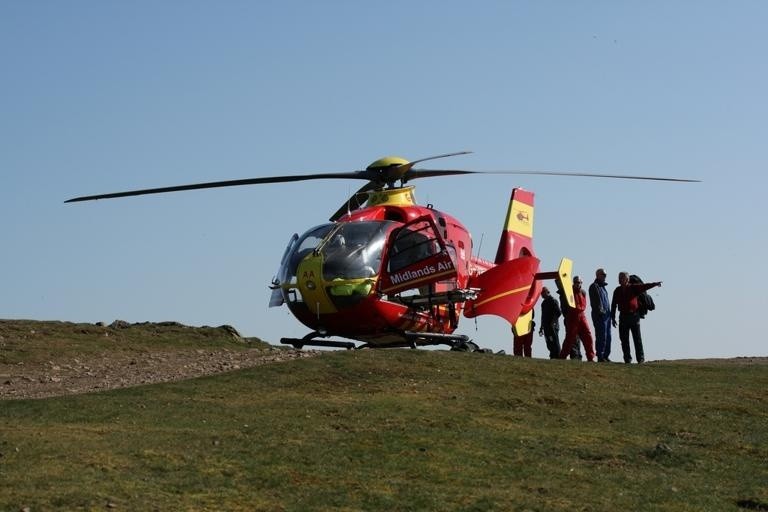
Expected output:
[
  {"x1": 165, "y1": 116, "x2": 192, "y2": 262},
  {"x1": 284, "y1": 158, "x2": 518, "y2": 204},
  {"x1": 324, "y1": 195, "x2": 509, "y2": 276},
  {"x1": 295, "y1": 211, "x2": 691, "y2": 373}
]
[{"x1": 61, "y1": 147, "x2": 709, "y2": 360}]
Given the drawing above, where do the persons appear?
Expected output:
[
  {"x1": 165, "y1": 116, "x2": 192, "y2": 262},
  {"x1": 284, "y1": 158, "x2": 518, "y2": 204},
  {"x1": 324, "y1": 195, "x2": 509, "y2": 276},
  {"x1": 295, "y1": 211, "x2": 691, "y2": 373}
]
[
  {"x1": 588, "y1": 268, "x2": 611, "y2": 363},
  {"x1": 611, "y1": 272, "x2": 664, "y2": 364},
  {"x1": 558, "y1": 276, "x2": 596, "y2": 362},
  {"x1": 539, "y1": 286, "x2": 561, "y2": 359},
  {"x1": 510, "y1": 306, "x2": 536, "y2": 358},
  {"x1": 569, "y1": 334, "x2": 582, "y2": 361}
]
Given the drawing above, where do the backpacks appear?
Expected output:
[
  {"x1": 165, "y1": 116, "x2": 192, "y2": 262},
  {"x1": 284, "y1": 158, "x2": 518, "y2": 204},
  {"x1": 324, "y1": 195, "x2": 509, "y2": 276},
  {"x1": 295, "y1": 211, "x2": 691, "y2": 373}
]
[{"x1": 628, "y1": 274, "x2": 655, "y2": 318}]
